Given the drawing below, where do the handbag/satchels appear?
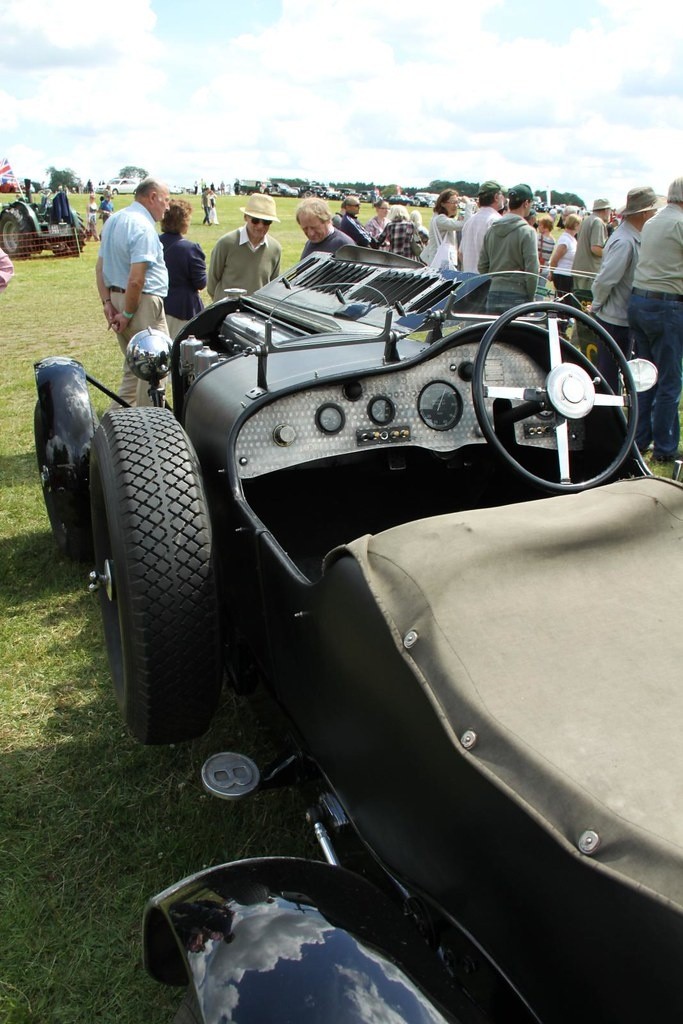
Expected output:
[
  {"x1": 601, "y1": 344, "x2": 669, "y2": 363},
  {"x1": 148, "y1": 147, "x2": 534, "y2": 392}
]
[
  {"x1": 411, "y1": 222, "x2": 424, "y2": 256},
  {"x1": 430, "y1": 213, "x2": 455, "y2": 271},
  {"x1": 543, "y1": 281, "x2": 558, "y2": 304},
  {"x1": 538, "y1": 252, "x2": 546, "y2": 273}
]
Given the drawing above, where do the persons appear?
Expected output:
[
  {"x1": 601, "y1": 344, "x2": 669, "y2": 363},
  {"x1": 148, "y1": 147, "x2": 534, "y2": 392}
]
[
  {"x1": 40, "y1": 179, "x2": 683, "y2": 464},
  {"x1": 0, "y1": 248, "x2": 14, "y2": 295}
]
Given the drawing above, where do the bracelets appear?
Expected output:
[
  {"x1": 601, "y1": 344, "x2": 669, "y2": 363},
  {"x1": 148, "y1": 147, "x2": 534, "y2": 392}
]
[
  {"x1": 123, "y1": 310, "x2": 133, "y2": 319},
  {"x1": 103, "y1": 298, "x2": 110, "y2": 305}
]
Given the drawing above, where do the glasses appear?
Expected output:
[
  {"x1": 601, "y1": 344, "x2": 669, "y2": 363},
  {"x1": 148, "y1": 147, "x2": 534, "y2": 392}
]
[
  {"x1": 347, "y1": 204, "x2": 359, "y2": 207},
  {"x1": 251, "y1": 218, "x2": 273, "y2": 226},
  {"x1": 442, "y1": 200, "x2": 461, "y2": 204}
]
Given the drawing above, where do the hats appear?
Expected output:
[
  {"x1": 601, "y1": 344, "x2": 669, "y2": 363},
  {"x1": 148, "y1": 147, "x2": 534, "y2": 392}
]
[
  {"x1": 508, "y1": 183, "x2": 540, "y2": 201},
  {"x1": 105, "y1": 191, "x2": 111, "y2": 196},
  {"x1": 620, "y1": 184, "x2": 667, "y2": 216},
  {"x1": 240, "y1": 193, "x2": 281, "y2": 223},
  {"x1": 476, "y1": 180, "x2": 504, "y2": 196},
  {"x1": 592, "y1": 198, "x2": 611, "y2": 210}
]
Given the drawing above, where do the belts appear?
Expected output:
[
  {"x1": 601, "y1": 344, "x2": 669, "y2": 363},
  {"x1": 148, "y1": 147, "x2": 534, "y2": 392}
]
[
  {"x1": 632, "y1": 289, "x2": 683, "y2": 301},
  {"x1": 109, "y1": 286, "x2": 145, "y2": 294}
]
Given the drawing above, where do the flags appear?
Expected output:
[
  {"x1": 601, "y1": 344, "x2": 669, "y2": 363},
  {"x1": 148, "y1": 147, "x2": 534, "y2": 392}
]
[{"x1": 0, "y1": 158, "x2": 15, "y2": 186}]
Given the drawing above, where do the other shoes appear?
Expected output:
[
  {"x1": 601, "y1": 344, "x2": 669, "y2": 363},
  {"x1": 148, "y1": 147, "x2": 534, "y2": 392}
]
[
  {"x1": 209, "y1": 222, "x2": 212, "y2": 226},
  {"x1": 641, "y1": 443, "x2": 654, "y2": 454},
  {"x1": 654, "y1": 451, "x2": 683, "y2": 461}
]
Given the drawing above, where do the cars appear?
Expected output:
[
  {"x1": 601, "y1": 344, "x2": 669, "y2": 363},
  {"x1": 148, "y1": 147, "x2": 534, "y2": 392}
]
[
  {"x1": 96, "y1": 178, "x2": 137, "y2": 194},
  {"x1": 30, "y1": 242, "x2": 683, "y2": 1020},
  {"x1": 234, "y1": 180, "x2": 583, "y2": 215}
]
